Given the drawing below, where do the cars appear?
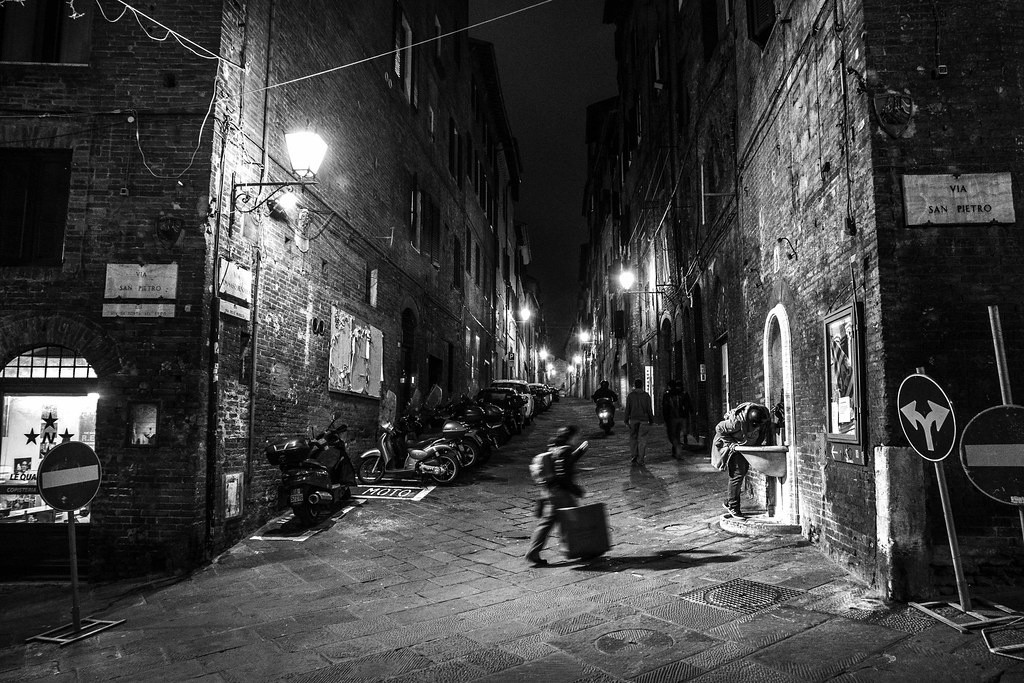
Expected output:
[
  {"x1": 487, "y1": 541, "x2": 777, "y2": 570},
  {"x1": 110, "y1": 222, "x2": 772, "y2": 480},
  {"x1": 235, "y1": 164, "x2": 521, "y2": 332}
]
[
  {"x1": 476, "y1": 387, "x2": 527, "y2": 430},
  {"x1": 528, "y1": 383, "x2": 561, "y2": 411}
]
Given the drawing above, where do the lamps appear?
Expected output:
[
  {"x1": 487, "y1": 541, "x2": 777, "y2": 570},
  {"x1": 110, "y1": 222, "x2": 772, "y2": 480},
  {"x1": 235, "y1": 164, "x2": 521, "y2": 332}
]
[
  {"x1": 620, "y1": 269, "x2": 665, "y2": 300},
  {"x1": 228, "y1": 131, "x2": 329, "y2": 236},
  {"x1": 510, "y1": 306, "x2": 530, "y2": 324}
]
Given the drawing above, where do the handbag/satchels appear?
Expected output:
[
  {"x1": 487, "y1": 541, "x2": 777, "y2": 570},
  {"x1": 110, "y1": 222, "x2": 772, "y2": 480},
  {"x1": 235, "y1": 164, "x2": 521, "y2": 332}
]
[{"x1": 556, "y1": 502, "x2": 611, "y2": 561}]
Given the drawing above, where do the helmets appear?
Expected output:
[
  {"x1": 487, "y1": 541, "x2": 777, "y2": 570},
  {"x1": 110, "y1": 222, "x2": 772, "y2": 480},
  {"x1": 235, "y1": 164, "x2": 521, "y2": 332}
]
[{"x1": 600, "y1": 380, "x2": 609, "y2": 388}]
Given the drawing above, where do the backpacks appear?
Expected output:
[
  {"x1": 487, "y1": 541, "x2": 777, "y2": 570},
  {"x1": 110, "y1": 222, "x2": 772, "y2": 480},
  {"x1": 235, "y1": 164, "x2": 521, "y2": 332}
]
[{"x1": 529, "y1": 452, "x2": 555, "y2": 487}]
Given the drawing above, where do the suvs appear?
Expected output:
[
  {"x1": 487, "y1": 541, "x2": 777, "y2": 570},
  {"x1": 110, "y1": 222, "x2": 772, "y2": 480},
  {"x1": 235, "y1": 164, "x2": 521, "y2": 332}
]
[{"x1": 491, "y1": 380, "x2": 537, "y2": 420}]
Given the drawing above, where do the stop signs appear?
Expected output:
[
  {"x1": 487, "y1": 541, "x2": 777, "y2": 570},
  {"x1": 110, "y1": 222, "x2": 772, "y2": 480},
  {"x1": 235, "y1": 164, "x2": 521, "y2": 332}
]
[
  {"x1": 35, "y1": 440, "x2": 102, "y2": 513},
  {"x1": 959, "y1": 404, "x2": 1024, "y2": 506}
]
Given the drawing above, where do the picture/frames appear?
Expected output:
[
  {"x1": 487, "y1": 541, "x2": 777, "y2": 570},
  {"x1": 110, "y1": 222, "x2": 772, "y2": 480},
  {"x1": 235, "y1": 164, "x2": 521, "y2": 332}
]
[{"x1": 822, "y1": 300, "x2": 865, "y2": 445}]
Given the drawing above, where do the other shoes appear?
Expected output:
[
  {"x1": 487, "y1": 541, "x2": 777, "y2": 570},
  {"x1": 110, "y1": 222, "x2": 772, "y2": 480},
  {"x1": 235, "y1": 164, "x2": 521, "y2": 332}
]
[
  {"x1": 525, "y1": 549, "x2": 547, "y2": 565},
  {"x1": 639, "y1": 463, "x2": 645, "y2": 467},
  {"x1": 631, "y1": 456, "x2": 637, "y2": 463}
]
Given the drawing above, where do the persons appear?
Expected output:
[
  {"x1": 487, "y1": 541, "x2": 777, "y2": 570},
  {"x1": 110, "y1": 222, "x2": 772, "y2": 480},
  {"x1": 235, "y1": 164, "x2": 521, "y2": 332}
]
[
  {"x1": 524, "y1": 424, "x2": 590, "y2": 565},
  {"x1": 593, "y1": 381, "x2": 617, "y2": 420},
  {"x1": 663, "y1": 381, "x2": 693, "y2": 455},
  {"x1": 623, "y1": 377, "x2": 656, "y2": 466},
  {"x1": 710, "y1": 399, "x2": 773, "y2": 521}
]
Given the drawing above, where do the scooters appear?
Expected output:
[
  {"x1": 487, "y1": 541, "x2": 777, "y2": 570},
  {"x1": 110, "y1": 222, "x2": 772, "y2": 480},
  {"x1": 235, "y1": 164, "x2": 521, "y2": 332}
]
[
  {"x1": 355, "y1": 382, "x2": 510, "y2": 486},
  {"x1": 263, "y1": 411, "x2": 358, "y2": 528},
  {"x1": 595, "y1": 398, "x2": 617, "y2": 436}
]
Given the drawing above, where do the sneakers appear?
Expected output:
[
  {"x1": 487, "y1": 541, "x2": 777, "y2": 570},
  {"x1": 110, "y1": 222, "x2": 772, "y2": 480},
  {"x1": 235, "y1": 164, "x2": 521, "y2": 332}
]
[{"x1": 723, "y1": 499, "x2": 746, "y2": 520}]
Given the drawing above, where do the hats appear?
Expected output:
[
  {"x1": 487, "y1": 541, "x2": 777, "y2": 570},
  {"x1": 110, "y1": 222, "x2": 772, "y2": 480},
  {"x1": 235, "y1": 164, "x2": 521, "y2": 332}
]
[{"x1": 556, "y1": 424, "x2": 579, "y2": 441}]
